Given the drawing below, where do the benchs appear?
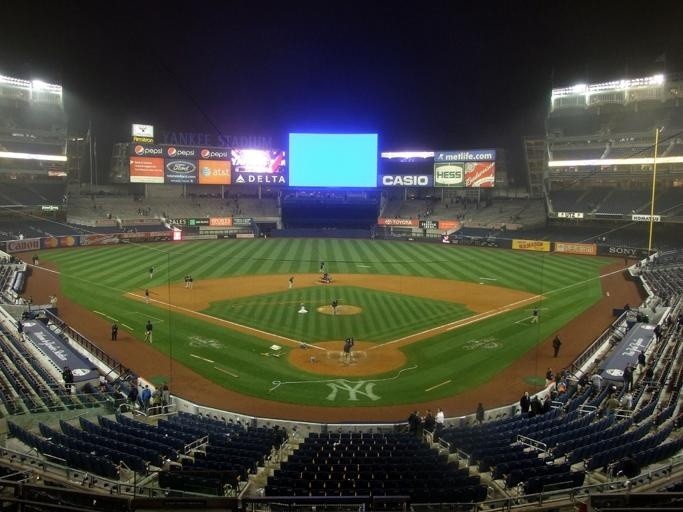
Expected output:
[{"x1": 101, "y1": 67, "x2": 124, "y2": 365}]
[
  {"x1": 1, "y1": 191, "x2": 282, "y2": 240},
  {"x1": 548, "y1": 108, "x2": 683, "y2": 185}
]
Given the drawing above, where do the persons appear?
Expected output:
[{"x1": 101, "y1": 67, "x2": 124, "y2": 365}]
[
  {"x1": 104, "y1": 213, "x2": 114, "y2": 220},
  {"x1": 47, "y1": 293, "x2": 57, "y2": 303},
  {"x1": 546, "y1": 334, "x2": 563, "y2": 360},
  {"x1": 625, "y1": 247, "x2": 683, "y2": 307},
  {"x1": 515, "y1": 362, "x2": 572, "y2": 419},
  {"x1": 181, "y1": 188, "x2": 279, "y2": 220},
  {"x1": 406, "y1": 404, "x2": 445, "y2": 439},
  {"x1": 61, "y1": 193, "x2": 68, "y2": 204},
  {"x1": 615, "y1": 452, "x2": 647, "y2": 482},
  {"x1": 591, "y1": 303, "x2": 681, "y2": 416},
  {"x1": 143, "y1": 289, "x2": 151, "y2": 304},
  {"x1": 14, "y1": 319, "x2": 26, "y2": 344},
  {"x1": 529, "y1": 303, "x2": 539, "y2": 326},
  {"x1": 182, "y1": 273, "x2": 194, "y2": 290},
  {"x1": 318, "y1": 261, "x2": 324, "y2": 278},
  {"x1": 341, "y1": 336, "x2": 354, "y2": 366},
  {"x1": 111, "y1": 321, "x2": 117, "y2": 341},
  {"x1": 61, "y1": 365, "x2": 74, "y2": 392},
  {"x1": 96, "y1": 374, "x2": 171, "y2": 417},
  {"x1": 329, "y1": 300, "x2": 339, "y2": 318},
  {"x1": 0, "y1": 253, "x2": 37, "y2": 306},
  {"x1": 17, "y1": 230, "x2": 24, "y2": 243},
  {"x1": 147, "y1": 265, "x2": 154, "y2": 280},
  {"x1": 473, "y1": 401, "x2": 488, "y2": 430},
  {"x1": 385, "y1": 191, "x2": 541, "y2": 225},
  {"x1": 135, "y1": 206, "x2": 149, "y2": 217},
  {"x1": 143, "y1": 320, "x2": 152, "y2": 344},
  {"x1": 318, "y1": 273, "x2": 333, "y2": 285},
  {"x1": 287, "y1": 274, "x2": 294, "y2": 291}
]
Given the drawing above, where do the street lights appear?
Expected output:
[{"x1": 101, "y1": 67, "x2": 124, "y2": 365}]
[{"x1": 647, "y1": 126, "x2": 665, "y2": 256}]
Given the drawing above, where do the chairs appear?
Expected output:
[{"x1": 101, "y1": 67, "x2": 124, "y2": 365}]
[
  {"x1": 1, "y1": 246, "x2": 126, "y2": 418},
  {"x1": 545, "y1": 246, "x2": 683, "y2": 435},
  {"x1": 0, "y1": 405, "x2": 682, "y2": 512},
  {"x1": 379, "y1": 185, "x2": 683, "y2": 246}
]
